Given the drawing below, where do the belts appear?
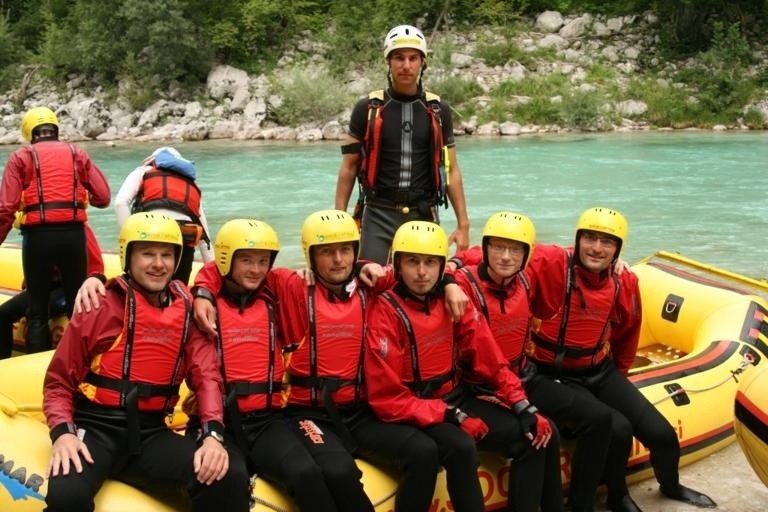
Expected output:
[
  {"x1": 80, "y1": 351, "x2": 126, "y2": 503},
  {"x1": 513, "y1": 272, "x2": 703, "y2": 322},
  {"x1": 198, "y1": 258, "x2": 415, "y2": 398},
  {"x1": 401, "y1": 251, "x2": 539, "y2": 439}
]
[{"x1": 375, "y1": 189, "x2": 434, "y2": 202}]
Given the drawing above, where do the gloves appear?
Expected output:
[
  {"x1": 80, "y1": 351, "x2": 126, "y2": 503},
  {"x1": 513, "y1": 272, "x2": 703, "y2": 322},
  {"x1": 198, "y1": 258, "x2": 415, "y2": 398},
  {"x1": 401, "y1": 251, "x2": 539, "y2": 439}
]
[
  {"x1": 512, "y1": 399, "x2": 553, "y2": 440},
  {"x1": 382, "y1": 24, "x2": 428, "y2": 58},
  {"x1": 443, "y1": 406, "x2": 489, "y2": 440}
]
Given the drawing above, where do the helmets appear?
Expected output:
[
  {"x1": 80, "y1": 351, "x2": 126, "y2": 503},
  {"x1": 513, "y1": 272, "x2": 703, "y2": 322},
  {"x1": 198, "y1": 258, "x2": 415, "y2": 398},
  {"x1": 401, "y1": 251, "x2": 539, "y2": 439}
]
[
  {"x1": 574, "y1": 207, "x2": 628, "y2": 258},
  {"x1": 21, "y1": 106, "x2": 60, "y2": 145},
  {"x1": 481, "y1": 211, "x2": 535, "y2": 270},
  {"x1": 119, "y1": 211, "x2": 184, "y2": 274},
  {"x1": 391, "y1": 220, "x2": 449, "y2": 281},
  {"x1": 301, "y1": 208, "x2": 361, "y2": 270},
  {"x1": 214, "y1": 218, "x2": 280, "y2": 277}
]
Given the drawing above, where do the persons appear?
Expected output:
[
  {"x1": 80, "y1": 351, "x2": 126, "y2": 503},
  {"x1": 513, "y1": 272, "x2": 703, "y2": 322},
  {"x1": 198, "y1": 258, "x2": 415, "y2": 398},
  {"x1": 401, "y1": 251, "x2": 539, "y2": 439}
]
[
  {"x1": 445, "y1": 205, "x2": 719, "y2": 511},
  {"x1": 0, "y1": 223, "x2": 103, "y2": 358},
  {"x1": 115, "y1": 147, "x2": 215, "y2": 286},
  {"x1": 294, "y1": 211, "x2": 635, "y2": 511},
  {"x1": 359, "y1": 220, "x2": 552, "y2": 512},
  {"x1": 335, "y1": 24, "x2": 469, "y2": 265},
  {"x1": 0, "y1": 107, "x2": 111, "y2": 352},
  {"x1": 69, "y1": 217, "x2": 386, "y2": 512},
  {"x1": 190, "y1": 208, "x2": 468, "y2": 512},
  {"x1": 41, "y1": 211, "x2": 233, "y2": 511}
]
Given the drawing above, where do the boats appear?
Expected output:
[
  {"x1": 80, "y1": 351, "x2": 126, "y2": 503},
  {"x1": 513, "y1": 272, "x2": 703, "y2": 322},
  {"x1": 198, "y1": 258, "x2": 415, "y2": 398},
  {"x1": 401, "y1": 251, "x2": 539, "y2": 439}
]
[
  {"x1": 0, "y1": 240, "x2": 204, "y2": 358},
  {"x1": 0, "y1": 250, "x2": 768, "y2": 511},
  {"x1": 733, "y1": 356, "x2": 768, "y2": 488}
]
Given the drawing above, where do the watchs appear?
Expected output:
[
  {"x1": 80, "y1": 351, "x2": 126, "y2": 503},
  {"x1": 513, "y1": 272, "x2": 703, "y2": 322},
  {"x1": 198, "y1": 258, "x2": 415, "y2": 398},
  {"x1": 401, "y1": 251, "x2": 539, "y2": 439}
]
[{"x1": 199, "y1": 430, "x2": 224, "y2": 445}]
